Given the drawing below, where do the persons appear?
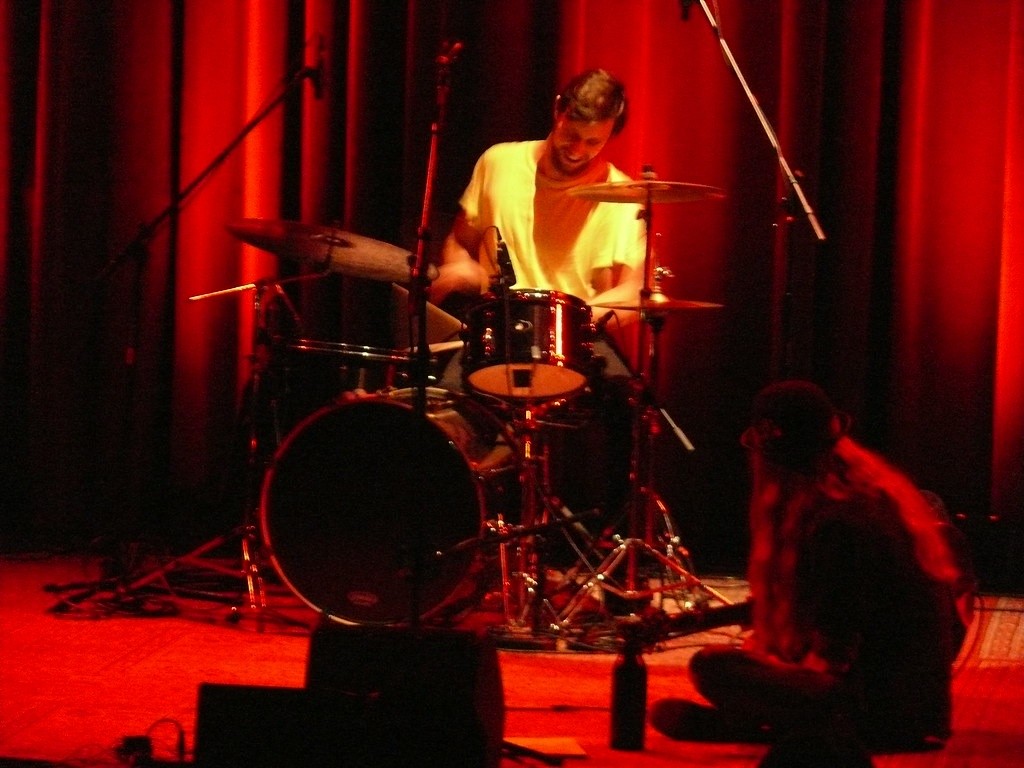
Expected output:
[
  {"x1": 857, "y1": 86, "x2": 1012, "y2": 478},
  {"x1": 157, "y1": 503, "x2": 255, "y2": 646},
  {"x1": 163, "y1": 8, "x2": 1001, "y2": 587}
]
[
  {"x1": 650, "y1": 381, "x2": 967, "y2": 754},
  {"x1": 431, "y1": 68, "x2": 649, "y2": 615}
]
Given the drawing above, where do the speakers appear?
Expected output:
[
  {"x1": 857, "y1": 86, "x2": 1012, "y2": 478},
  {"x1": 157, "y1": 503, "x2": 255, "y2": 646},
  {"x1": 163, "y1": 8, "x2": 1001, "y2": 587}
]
[{"x1": 303, "y1": 622, "x2": 502, "y2": 768}]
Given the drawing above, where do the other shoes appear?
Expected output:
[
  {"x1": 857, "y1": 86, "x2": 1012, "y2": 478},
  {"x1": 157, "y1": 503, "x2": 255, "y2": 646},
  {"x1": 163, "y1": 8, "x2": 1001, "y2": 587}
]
[{"x1": 652, "y1": 699, "x2": 764, "y2": 742}]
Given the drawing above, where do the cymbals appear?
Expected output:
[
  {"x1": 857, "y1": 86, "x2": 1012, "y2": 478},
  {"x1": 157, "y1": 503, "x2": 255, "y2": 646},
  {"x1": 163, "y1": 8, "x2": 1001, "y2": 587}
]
[
  {"x1": 223, "y1": 215, "x2": 438, "y2": 282},
  {"x1": 595, "y1": 295, "x2": 724, "y2": 314},
  {"x1": 568, "y1": 179, "x2": 729, "y2": 203}
]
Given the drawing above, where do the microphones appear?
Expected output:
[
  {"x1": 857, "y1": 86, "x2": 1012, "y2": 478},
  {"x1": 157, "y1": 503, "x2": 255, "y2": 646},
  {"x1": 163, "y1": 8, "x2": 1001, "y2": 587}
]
[
  {"x1": 496, "y1": 228, "x2": 516, "y2": 287},
  {"x1": 431, "y1": 549, "x2": 450, "y2": 575},
  {"x1": 592, "y1": 311, "x2": 616, "y2": 340},
  {"x1": 314, "y1": 37, "x2": 325, "y2": 99}
]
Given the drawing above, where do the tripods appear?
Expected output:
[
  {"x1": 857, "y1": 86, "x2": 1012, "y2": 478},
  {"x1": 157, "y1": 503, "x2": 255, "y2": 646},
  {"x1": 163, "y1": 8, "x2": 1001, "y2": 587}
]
[
  {"x1": 460, "y1": 313, "x2": 747, "y2": 636},
  {"x1": 53, "y1": 262, "x2": 334, "y2": 622}
]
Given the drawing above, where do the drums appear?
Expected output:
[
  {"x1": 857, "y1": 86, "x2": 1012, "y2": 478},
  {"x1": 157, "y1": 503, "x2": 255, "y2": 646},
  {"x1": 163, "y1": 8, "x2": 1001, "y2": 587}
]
[
  {"x1": 458, "y1": 287, "x2": 595, "y2": 399},
  {"x1": 259, "y1": 384, "x2": 520, "y2": 630},
  {"x1": 269, "y1": 336, "x2": 419, "y2": 436}
]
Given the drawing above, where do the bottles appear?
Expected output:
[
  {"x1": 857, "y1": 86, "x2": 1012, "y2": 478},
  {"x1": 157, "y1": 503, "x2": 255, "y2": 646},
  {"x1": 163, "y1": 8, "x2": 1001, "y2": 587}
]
[{"x1": 612, "y1": 629, "x2": 646, "y2": 750}]
